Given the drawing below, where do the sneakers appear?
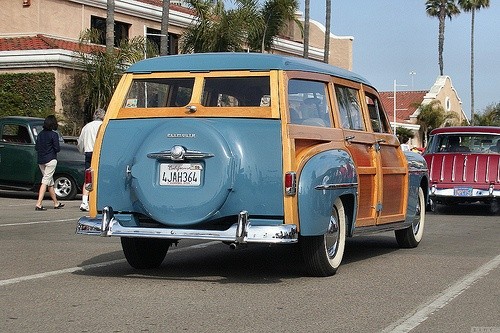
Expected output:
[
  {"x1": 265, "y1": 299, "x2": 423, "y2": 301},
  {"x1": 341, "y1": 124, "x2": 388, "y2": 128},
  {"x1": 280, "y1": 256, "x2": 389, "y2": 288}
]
[{"x1": 79, "y1": 203, "x2": 89, "y2": 212}]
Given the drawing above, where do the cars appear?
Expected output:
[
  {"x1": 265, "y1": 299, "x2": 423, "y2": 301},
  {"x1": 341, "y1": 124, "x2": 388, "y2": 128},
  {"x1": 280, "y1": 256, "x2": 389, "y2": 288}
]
[
  {"x1": 0, "y1": 117, "x2": 86, "y2": 201},
  {"x1": 74, "y1": 53, "x2": 430, "y2": 277},
  {"x1": 420, "y1": 127, "x2": 499, "y2": 214}
]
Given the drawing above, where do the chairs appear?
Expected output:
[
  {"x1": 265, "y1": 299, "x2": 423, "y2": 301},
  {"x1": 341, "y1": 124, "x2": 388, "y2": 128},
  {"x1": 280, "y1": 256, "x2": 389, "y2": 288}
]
[
  {"x1": 366, "y1": 102, "x2": 381, "y2": 133},
  {"x1": 300, "y1": 99, "x2": 321, "y2": 121}
]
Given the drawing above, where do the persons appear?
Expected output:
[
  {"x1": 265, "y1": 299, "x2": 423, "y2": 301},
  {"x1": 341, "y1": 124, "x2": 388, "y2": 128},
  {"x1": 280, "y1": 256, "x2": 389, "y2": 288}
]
[
  {"x1": 79, "y1": 108, "x2": 105, "y2": 212},
  {"x1": 35, "y1": 114, "x2": 65, "y2": 211},
  {"x1": 400, "y1": 137, "x2": 416, "y2": 152},
  {"x1": 443, "y1": 136, "x2": 471, "y2": 152}
]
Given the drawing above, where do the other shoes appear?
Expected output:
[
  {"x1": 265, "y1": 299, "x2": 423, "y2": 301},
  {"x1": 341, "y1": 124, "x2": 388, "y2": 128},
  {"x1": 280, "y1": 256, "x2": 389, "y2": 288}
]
[
  {"x1": 54, "y1": 202, "x2": 65, "y2": 209},
  {"x1": 34, "y1": 205, "x2": 47, "y2": 211}
]
